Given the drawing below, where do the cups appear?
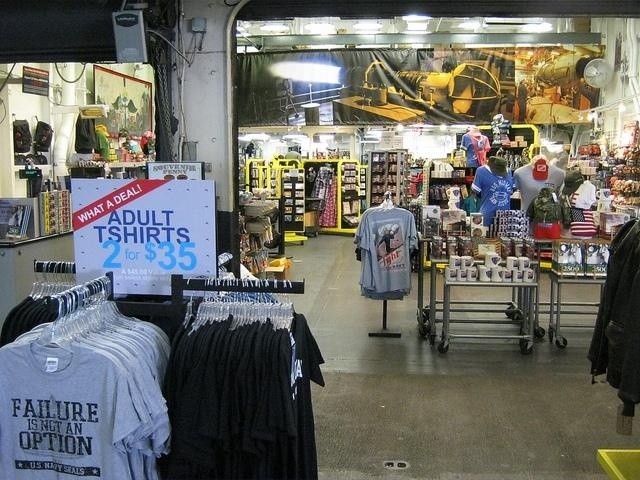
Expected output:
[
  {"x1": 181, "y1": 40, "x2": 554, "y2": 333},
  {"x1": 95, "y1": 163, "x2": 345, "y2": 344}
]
[
  {"x1": 597, "y1": 188, "x2": 612, "y2": 202},
  {"x1": 445, "y1": 251, "x2": 537, "y2": 283}
]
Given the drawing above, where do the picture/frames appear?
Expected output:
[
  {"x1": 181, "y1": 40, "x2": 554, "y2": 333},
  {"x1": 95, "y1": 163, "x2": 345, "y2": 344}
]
[{"x1": 93, "y1": 64, "x2": 152, "y2": 140}]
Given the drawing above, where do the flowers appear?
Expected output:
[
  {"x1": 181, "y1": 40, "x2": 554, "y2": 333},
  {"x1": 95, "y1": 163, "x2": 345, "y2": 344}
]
[{"x1": 112, "y1": 87, "x2": 138, "y2": 128}]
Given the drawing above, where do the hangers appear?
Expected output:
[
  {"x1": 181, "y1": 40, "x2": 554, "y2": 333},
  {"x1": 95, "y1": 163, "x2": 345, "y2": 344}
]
[
  {"x1": 182, "y1": 275, "x2": 296, "y2": 339},
  {"x1": 29, "y1": 260, "x2": 135, "y2": 354}
]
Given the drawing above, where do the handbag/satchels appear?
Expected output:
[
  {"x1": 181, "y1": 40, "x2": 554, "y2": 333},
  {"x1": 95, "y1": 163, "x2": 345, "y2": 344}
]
[
  {"x1": 569, "y1": 207, "x2": 597, "y2": 236},
  {"x1": 535, "y1": 223, "x2": 561, "y2": 239},
  {"x1": 118, "y1": 128, "x2": 155, "y2": 155},
  {"x1": 13, "y1": 113, "x2": 54, "y2": 165}
]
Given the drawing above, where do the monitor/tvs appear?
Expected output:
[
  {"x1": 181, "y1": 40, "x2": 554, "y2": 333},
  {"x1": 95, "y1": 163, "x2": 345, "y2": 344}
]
[{"x1": 22, "y1": 66, "x2": 49, "y2": 97}]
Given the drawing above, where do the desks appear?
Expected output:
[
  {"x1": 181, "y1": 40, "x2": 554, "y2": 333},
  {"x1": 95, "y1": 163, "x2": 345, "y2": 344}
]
[
  {"x1": 68, "y1": 162, "x2": 147, "y2": 178},
  {"x1": 0, "y1": 229, "x2": 74, "y2": 340}
]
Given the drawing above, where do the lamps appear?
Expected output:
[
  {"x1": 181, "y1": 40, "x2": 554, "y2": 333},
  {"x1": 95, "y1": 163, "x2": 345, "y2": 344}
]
[
  {"x1": 78, "y1": 104, "x2": 109, "y2": 120},
  {"x1": 574, "y1": 57, "x2": 614, "y2": 89}
]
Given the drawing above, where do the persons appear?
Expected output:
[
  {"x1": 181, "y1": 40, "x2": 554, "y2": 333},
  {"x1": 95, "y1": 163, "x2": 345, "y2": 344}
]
[
  {"x1": 510, "y1": 154, "x2": 566, "y2": 221},
  {"x1": 487, "y1": 114, "x2": 517, "y2": 156},
  {"x1": 461, "y1": 122, "x2": 489, "y2": 167},
  {"x1": 469, "y1": 155, "x2": 517, "y2": 226}
]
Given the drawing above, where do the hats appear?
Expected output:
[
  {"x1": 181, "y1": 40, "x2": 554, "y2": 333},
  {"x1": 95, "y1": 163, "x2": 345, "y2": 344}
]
[
  {"x1": 562, "y1": 172, "x2": 583, "y2": 194},
  {"x1": 488, "y1": 157, "x2": 507, "y2": 176},
  {"x1": 531, "y1": 154, "x2": 548, "y2": 180}
]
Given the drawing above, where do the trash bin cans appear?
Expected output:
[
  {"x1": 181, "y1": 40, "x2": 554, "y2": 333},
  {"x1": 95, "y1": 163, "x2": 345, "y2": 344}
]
[{"x1": 303, "y1": 198, "x2": 323, "y2": 237}]
